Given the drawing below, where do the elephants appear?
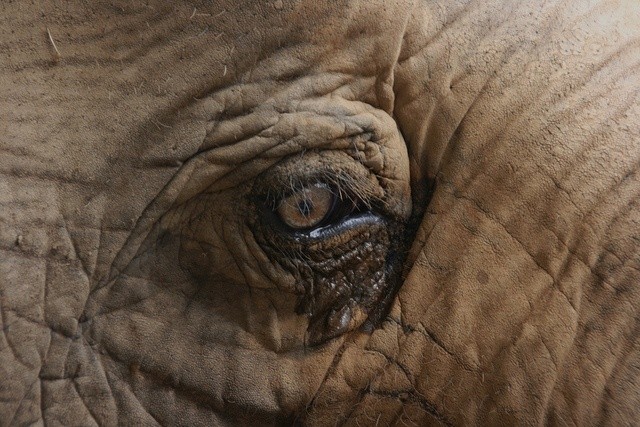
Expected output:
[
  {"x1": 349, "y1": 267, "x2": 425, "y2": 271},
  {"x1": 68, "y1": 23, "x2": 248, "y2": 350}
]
[{"x1": 0, "y1": 0, "x2": 640, "y2": 424}]
